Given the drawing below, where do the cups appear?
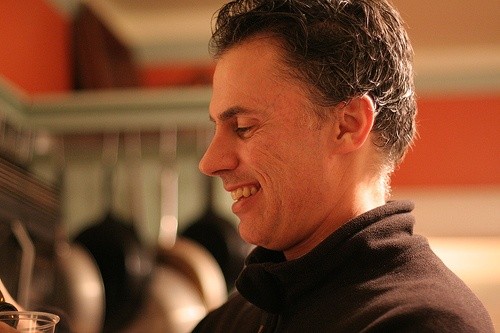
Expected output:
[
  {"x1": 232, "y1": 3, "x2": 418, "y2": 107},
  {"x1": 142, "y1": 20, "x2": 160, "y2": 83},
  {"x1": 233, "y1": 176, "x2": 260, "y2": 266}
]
[{"x1": 0, "y1": 311, "x2": 60, "y2": 333}]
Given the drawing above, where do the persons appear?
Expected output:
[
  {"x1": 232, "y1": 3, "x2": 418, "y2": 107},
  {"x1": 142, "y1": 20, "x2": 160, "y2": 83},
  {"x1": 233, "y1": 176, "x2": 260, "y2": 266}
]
[{"x1": 0, "y1": 0, "x2": 495, "y2": 333}]
